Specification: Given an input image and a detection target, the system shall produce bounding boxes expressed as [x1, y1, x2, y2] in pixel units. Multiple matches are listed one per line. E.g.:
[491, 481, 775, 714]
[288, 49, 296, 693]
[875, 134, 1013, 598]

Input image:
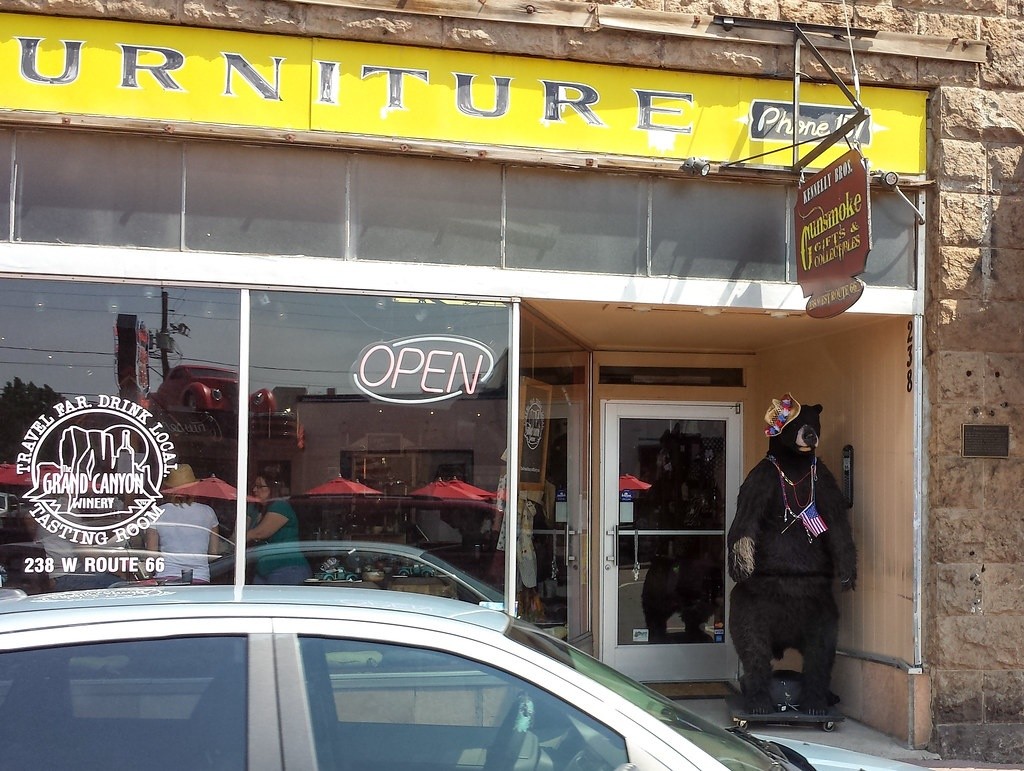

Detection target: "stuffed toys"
[728, 395, 855, 714]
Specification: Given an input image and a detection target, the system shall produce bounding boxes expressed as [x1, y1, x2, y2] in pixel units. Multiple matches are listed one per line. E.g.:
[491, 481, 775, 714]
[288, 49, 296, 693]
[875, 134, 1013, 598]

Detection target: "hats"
[162, 464, 196, 487]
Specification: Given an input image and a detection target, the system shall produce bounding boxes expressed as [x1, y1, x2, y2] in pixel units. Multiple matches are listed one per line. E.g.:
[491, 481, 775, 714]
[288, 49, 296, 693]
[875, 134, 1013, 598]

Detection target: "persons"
[226, 471, 312, 586]
[139, 464, 219, 585]
[497, 450, 544, 617]
[26, 474, 123, 592]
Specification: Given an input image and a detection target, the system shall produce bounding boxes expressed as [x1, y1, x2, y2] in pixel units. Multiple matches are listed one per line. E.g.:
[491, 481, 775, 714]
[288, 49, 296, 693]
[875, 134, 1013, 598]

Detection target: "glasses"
[253, 484, 269, 490]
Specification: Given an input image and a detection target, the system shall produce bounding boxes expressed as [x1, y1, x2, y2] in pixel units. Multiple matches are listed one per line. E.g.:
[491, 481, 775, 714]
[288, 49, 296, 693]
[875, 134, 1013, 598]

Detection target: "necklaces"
[764, 455, 818, 544]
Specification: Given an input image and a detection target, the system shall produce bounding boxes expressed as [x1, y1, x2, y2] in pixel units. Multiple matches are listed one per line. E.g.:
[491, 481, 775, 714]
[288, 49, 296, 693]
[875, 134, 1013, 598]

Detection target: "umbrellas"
[618, 474, 652, 490]
[160, 479, 259, 502]
[408, 480, 495, 500]
[0, 463, 101, 491]
[304, 478, 378, 495]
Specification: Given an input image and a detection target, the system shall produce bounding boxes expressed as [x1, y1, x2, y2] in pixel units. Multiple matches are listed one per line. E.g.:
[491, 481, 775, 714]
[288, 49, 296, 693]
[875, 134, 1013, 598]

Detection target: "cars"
[0, 492, 21, 514]
[0, 585, 931, 771]
[314, 567, 359, 582]
[149, 364, 277, 412]
[107, 539, 524, 621]
[397, 564, 438, 578]
[11, 502, 25, 510]
[1, 522, 236, 589]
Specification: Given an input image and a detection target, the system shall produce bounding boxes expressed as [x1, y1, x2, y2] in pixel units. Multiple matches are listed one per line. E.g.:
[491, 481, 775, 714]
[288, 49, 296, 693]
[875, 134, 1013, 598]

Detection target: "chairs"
[0, 657, 75, 771]
[0, 545, 50, 595]
[192, 661, 247, 771]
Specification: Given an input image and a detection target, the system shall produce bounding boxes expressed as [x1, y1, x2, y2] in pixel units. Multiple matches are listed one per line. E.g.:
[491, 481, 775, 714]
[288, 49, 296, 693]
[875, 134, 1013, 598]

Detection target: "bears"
[632, 428, 725, 645]
[727, 393, 859, 714]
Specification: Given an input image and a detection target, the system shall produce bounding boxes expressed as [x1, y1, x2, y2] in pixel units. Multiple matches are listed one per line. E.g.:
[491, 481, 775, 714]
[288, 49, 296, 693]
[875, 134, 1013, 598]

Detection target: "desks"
[301, 574, 457, 599]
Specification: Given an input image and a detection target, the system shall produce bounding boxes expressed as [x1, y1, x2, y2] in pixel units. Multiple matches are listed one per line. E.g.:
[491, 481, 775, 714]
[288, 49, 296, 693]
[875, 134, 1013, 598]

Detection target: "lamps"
[681, 156, 710, 178]
[874, 171, 901, 191]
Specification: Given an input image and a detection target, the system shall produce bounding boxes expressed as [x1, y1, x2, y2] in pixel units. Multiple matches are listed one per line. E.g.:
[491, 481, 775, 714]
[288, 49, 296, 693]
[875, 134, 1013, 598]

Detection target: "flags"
[800, 503, 828, 538]
